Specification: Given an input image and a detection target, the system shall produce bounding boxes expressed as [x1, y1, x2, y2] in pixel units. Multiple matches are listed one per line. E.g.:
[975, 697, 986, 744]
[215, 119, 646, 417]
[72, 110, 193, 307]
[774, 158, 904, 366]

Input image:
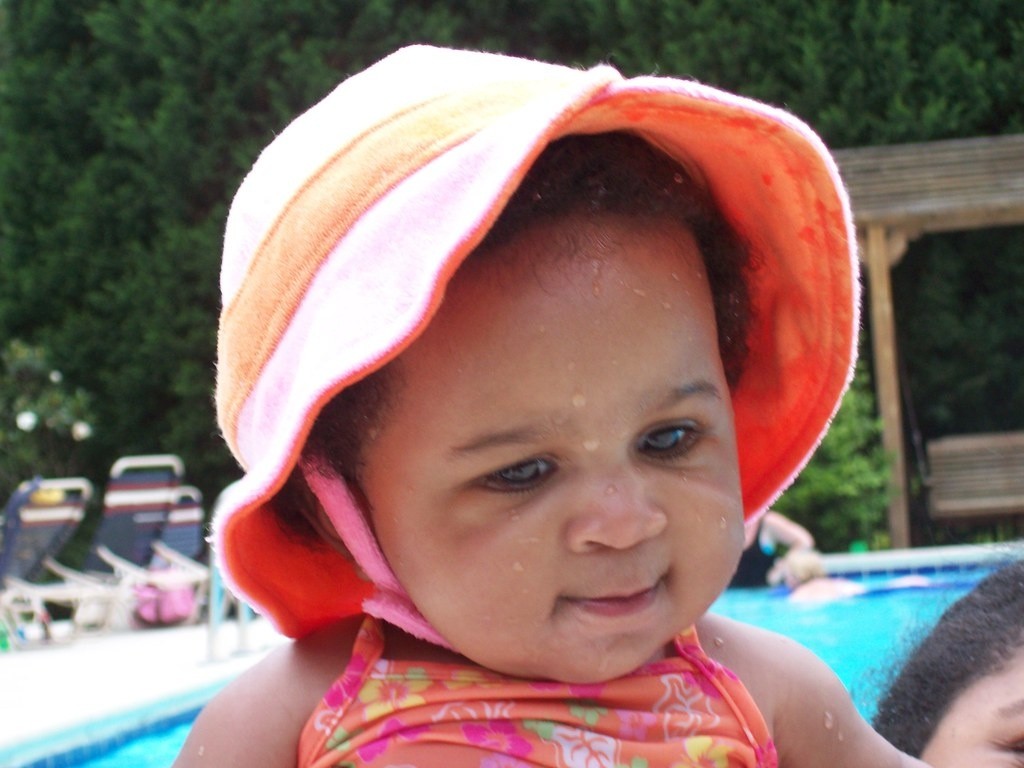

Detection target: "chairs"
[78, 453, 211, 633]
[164, 485, 205, 560]
[0, 477, 116, 652]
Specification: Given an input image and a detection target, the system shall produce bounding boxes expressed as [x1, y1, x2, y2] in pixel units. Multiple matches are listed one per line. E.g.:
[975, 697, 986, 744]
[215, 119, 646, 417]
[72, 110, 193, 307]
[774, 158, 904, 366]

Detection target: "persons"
[168, 43, 934, 768]
[727, 511, 929, 601]
[872, 560, 1024, 768]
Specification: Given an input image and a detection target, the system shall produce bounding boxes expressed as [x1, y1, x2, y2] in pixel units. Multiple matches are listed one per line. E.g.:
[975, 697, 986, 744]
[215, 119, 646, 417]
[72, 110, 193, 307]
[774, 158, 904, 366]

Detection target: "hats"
[210, 44, 861, 639]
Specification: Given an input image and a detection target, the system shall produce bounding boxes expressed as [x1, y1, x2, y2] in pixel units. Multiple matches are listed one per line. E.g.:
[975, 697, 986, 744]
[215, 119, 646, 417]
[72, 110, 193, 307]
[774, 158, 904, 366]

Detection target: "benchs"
[926, 430, 1024, 543]
